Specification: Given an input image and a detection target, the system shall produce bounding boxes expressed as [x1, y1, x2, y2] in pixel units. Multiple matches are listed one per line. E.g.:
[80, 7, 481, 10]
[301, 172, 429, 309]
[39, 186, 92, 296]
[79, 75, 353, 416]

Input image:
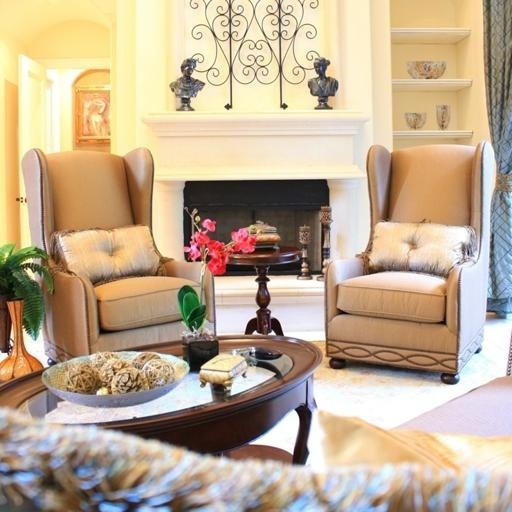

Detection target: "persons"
[307, 56, 339, 98]
[168, 58, 205, 100]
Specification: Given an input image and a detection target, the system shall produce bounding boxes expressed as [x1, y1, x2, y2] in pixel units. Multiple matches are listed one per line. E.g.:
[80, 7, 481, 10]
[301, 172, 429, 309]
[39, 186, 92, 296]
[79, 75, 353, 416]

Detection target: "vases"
[198, 262, 218, 341]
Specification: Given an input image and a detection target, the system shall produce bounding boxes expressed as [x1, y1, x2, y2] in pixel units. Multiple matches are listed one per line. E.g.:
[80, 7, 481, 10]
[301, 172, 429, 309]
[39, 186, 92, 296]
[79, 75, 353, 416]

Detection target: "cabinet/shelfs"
[390, 26, 473, 140]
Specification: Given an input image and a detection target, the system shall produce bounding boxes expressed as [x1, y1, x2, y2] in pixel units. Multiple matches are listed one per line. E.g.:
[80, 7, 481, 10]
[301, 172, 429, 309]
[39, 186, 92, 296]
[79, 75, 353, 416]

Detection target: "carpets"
[216, 303, 512, 470]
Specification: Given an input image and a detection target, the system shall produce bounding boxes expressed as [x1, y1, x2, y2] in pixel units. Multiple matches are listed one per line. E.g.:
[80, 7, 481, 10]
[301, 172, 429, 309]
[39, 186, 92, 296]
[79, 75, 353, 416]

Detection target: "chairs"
[18, 145, 216, 365]
[324, 140, 496, 386]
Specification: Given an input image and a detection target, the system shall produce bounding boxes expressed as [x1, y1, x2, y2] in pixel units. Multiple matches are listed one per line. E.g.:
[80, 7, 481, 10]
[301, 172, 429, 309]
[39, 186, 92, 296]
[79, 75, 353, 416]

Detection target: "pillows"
[50, 223, 167, 284]
[368, 220, 476, 278]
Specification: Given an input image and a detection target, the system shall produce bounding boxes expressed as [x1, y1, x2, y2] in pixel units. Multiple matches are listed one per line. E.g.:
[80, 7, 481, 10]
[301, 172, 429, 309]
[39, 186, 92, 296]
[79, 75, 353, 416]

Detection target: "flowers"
[178, 204, 257, 336]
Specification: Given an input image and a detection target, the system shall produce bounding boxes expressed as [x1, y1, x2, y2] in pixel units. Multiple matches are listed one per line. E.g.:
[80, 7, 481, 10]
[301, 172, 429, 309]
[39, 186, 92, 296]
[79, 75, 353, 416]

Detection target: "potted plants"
[0, 240, 55, 354]
[178, 285, 220, 373]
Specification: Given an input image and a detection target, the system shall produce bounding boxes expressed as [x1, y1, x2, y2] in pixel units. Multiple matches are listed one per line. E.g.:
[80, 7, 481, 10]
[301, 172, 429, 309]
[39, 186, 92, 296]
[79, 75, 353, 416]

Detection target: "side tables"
[228, 247, 302, 335]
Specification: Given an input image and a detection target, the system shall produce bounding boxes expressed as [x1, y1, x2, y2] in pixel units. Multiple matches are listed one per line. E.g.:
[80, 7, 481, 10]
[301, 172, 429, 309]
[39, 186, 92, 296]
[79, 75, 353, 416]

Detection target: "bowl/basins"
[40, 348, 191, 409]
